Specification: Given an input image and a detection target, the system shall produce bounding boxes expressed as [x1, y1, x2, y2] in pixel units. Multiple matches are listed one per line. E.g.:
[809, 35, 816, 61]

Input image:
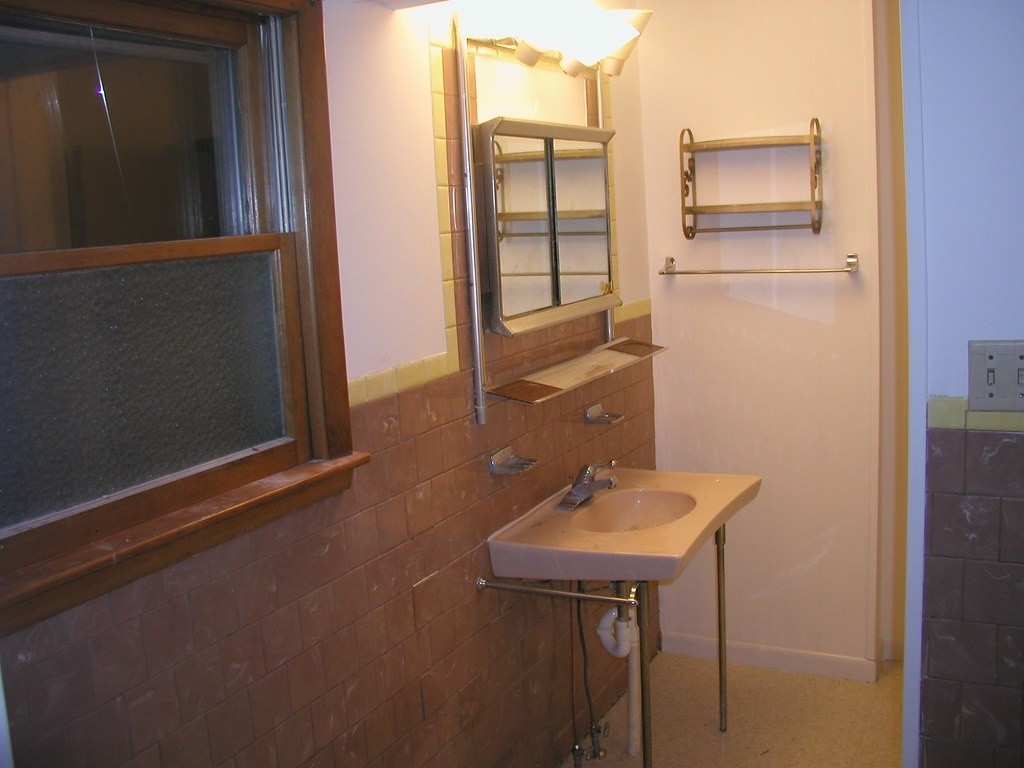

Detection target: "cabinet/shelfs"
[493, 141, 606, 242]
[679, 117, 822, 240]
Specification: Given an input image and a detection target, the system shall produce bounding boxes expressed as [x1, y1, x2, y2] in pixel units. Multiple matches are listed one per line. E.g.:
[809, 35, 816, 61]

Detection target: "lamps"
[515, 0, 654, 81]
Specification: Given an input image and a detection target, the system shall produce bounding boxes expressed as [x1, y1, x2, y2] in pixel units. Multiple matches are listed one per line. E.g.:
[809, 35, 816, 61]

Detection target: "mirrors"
[474, 115, 625, 338]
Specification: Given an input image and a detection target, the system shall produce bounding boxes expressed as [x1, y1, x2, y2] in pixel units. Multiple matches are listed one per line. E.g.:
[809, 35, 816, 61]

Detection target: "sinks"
[486, 466, 763, 581]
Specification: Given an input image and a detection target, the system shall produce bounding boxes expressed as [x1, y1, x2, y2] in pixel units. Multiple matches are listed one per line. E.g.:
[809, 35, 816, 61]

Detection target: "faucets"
[556, 460, 619, 512]
